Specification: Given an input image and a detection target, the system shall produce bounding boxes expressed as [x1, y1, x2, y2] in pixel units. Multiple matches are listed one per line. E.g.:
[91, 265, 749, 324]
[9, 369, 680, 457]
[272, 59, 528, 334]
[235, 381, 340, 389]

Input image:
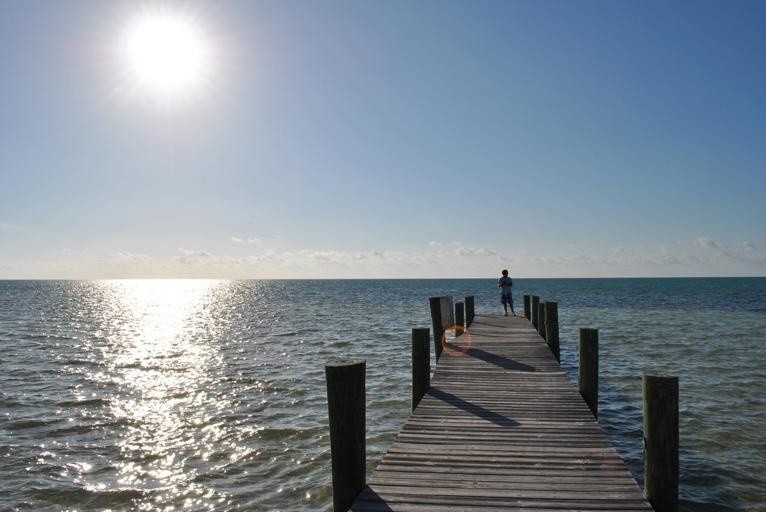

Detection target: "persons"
[497, 269, 516, 316]
